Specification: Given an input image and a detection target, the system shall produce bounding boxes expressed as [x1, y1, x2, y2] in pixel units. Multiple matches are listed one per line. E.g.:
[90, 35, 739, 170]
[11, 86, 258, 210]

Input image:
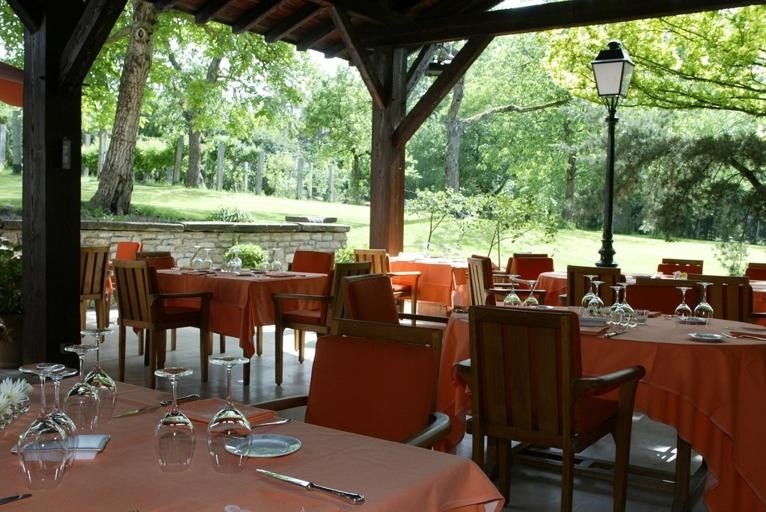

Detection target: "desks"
[442, 304, 766, 504]
[0, 363, 504, 512]
[152, 267, 328, 386]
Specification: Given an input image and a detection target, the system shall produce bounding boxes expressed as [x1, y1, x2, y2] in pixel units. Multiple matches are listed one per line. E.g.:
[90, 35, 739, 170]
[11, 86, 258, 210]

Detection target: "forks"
[721, 331, 765, 341]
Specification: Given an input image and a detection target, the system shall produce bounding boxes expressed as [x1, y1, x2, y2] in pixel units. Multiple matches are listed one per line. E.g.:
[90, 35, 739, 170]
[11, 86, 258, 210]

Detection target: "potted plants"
[0, 239, 26, 370]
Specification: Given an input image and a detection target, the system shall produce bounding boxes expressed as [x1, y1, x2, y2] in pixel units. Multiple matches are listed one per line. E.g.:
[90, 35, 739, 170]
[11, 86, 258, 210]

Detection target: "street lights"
[589, 39, 634, 267]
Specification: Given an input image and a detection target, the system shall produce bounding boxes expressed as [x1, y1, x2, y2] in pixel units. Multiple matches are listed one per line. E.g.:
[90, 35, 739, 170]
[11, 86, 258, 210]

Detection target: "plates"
[689, 332, 725, 343]
[223, 433, 302, 460]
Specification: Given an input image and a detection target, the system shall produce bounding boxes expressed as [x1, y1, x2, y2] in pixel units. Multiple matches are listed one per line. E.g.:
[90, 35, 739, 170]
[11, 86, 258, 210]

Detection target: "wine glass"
[578, 273, 638, 331]
[153, 366, 196, 474]
[190, 246, 282, 275]
[694, 282, 715, 329]
[205, 354, 253, 476]
[521, 281, 541, 307]
[503, 275, 523, 307]
[15, 327, 116, 491]
[674, 285, 693, 330]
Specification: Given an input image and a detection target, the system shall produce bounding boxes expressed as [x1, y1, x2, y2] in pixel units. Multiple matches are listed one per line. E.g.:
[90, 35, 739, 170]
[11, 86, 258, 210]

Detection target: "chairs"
[305, 320, 450, 452]
[457, 306, 646, 512]
[82, 242, 766, 387]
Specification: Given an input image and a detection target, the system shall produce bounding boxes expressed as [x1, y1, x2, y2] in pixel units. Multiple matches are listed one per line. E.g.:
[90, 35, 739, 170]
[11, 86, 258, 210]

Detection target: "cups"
[634, 307, 649, 325]
[680, 273, 687, 281]
[674, 272, 681, 280]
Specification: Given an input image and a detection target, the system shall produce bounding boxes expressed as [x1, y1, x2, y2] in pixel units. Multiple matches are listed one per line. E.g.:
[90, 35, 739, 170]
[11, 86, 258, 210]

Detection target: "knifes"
[596, 326, 626, 337]
[0, 494, 32, 506]
[111, 393, 201, 420]
[256, 467, 366, 506]
[182, 270, 218, 277]
